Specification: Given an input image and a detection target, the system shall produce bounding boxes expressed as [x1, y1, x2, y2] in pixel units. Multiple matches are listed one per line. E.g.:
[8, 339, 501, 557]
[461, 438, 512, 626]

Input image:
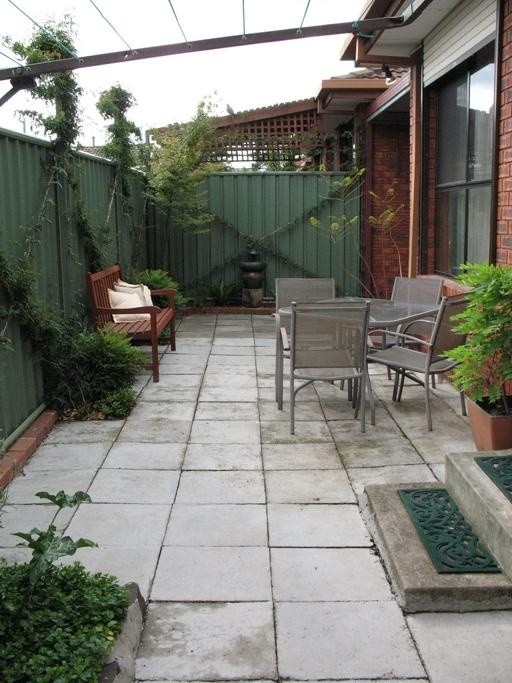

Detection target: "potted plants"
[441, 262, 512, 450]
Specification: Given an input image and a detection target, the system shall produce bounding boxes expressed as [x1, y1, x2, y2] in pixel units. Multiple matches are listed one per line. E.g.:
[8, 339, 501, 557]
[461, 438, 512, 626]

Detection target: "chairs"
[354, 275, 445, 388]
[277, 302, 368, 436]
[351, 290, 480, 434]
[275, 274, 347, 394]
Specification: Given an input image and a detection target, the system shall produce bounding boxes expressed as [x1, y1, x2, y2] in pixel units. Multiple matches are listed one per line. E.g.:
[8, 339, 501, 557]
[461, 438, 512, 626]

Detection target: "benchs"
[88, 262, 178, 382]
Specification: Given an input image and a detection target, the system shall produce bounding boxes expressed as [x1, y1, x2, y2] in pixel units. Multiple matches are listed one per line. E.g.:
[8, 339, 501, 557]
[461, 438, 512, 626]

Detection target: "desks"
[271, 293, 450, 412]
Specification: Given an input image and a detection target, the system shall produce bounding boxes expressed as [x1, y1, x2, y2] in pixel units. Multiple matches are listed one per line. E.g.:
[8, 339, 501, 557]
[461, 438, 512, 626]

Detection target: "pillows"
[106, 276, 156, 325]
